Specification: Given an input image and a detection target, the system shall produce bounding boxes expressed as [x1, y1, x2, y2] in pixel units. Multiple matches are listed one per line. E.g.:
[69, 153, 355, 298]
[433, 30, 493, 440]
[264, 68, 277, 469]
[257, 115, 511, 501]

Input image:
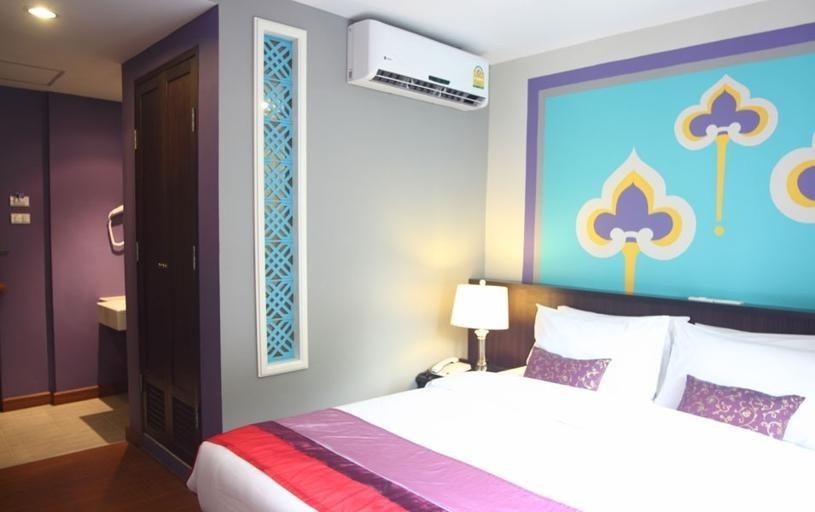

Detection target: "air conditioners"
[346, 19, 490, 112]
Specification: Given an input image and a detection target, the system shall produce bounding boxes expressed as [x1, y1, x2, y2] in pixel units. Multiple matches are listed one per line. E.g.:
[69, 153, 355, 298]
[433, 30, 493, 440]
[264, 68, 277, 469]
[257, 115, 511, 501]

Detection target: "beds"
[186, 280, 815, 512]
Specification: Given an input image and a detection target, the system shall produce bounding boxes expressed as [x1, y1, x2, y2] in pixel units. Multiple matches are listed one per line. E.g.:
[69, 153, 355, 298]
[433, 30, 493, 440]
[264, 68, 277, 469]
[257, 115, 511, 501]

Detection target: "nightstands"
[416, 356, 508, 388]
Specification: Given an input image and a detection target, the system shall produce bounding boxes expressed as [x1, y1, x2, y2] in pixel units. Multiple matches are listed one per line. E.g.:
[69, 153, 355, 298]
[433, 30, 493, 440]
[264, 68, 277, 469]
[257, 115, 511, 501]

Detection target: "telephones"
[430, 357, 472, 376]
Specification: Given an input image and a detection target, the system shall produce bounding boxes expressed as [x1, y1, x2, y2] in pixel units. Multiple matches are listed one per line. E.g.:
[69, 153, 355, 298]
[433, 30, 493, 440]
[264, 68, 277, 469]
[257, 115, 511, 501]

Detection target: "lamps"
[451, 280, 510, 372]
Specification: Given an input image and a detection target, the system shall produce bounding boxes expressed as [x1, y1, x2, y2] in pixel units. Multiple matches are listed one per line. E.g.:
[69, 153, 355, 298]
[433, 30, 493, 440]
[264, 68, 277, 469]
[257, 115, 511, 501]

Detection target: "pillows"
[526, 302, 671, 406]
[522, 346, 612, 393]
[692, 320, 815, 352]
[654, 320, 815, 448]
[675, 373, 805, 444]
[556, 303, 691, 397]
